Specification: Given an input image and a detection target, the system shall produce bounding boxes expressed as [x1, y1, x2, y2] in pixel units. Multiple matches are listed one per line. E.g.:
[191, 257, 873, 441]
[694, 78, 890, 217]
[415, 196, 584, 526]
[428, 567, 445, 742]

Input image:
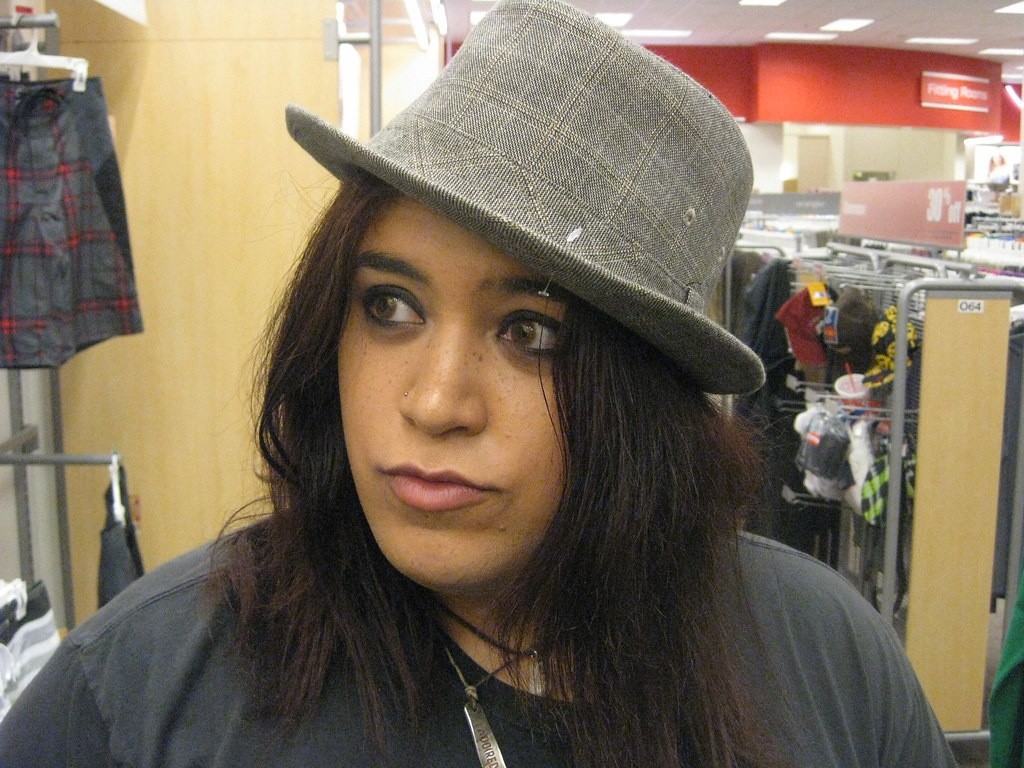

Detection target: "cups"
[834, 373, 872, 423]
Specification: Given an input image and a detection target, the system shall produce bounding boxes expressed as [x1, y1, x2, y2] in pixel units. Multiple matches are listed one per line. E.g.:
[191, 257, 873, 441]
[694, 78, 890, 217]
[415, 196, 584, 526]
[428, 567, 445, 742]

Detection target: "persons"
[0, 1, 965, 768]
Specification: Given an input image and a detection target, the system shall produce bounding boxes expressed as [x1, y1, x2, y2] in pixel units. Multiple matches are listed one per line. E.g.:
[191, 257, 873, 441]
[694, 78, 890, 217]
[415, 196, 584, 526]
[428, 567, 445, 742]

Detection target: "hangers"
[0, 577, 42, 638]
[108, 453, 128, 526]
[0, 11, 89, 93]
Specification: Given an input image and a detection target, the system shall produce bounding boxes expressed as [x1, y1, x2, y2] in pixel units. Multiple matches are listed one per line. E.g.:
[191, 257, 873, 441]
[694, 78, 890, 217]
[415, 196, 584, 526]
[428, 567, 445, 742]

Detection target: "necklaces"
[436, 601, 559, 767]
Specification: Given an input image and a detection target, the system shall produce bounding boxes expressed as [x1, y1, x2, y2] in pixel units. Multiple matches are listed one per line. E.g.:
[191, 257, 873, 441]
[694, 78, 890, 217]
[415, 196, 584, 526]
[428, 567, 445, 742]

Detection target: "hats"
[813, 291, 877, 373]
[864, 305, 917, 389]
[281, 1, 765, 394]
[773, 288, 839, 367]
